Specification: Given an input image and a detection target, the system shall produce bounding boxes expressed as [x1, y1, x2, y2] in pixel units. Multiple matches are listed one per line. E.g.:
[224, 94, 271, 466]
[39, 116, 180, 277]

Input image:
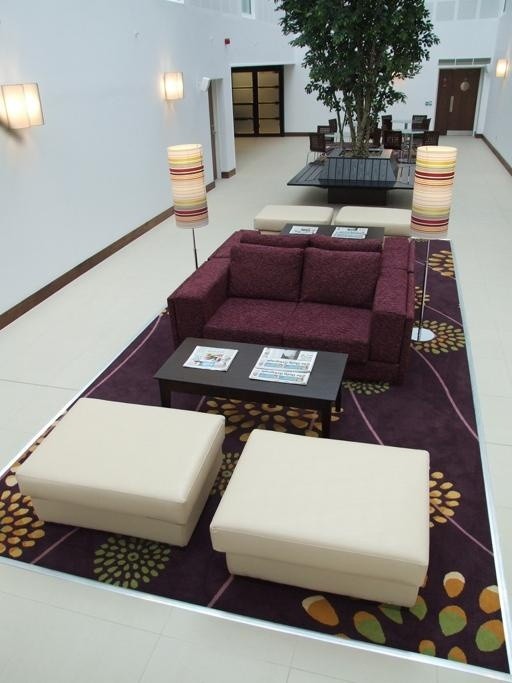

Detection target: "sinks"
[166, 242, 413, 383]
[208, 229, 416, 273]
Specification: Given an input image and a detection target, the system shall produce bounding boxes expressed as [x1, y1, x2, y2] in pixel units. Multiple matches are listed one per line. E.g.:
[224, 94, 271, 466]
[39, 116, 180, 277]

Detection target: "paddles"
[0, 235, 509, 681]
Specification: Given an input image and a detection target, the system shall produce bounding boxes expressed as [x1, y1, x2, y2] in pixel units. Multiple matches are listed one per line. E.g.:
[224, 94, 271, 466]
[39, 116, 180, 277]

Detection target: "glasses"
[164, 71, 184, 103]
[410, 145, 457, 340]
[166, 143, 210, 271]
[495, 59, 507, 78]
[0, 82, 45, 132]
[494, 58, 507, 80]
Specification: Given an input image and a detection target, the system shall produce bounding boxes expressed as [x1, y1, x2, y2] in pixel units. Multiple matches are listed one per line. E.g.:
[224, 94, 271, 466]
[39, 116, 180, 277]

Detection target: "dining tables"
[273, 0, 441, 160]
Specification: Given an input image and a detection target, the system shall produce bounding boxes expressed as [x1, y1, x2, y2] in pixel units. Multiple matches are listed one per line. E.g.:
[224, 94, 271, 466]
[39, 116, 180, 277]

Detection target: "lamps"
[305, 114, 439, 165]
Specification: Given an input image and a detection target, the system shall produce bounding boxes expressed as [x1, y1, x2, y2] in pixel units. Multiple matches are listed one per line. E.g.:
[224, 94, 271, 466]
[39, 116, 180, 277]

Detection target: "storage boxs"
[287, 149, 415, 204]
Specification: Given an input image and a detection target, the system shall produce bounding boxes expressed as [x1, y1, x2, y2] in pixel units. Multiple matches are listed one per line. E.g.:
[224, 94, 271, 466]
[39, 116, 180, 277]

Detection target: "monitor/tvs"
[331, 226, 368, 239]
[182, 345, 239, 372]
[289, 224, 319, 235]
[249, 347, 317, 385]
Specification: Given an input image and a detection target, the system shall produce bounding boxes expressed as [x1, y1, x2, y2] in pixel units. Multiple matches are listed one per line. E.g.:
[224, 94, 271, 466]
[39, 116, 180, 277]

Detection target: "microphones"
[254, 205, 333, 231]
[17, 397, 225, 550]
[334, 206, 412, 236]
[209, 428, 432, 611]
